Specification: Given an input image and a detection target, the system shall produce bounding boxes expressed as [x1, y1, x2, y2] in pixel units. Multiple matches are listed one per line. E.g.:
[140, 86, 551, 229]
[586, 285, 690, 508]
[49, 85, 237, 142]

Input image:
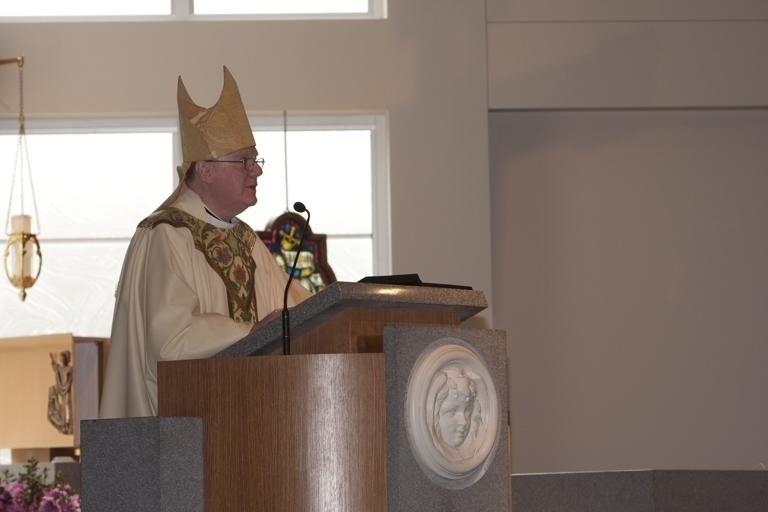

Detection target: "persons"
[98, 147, 314, 421]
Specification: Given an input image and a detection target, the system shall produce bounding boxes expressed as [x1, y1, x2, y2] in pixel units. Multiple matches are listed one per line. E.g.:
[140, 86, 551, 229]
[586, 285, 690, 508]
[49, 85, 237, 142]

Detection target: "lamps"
[0, 56, 42, 302]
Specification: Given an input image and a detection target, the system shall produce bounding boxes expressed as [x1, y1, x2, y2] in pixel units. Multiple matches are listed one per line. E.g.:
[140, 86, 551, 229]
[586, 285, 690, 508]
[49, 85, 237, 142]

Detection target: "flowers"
[2, 456, 80, 512]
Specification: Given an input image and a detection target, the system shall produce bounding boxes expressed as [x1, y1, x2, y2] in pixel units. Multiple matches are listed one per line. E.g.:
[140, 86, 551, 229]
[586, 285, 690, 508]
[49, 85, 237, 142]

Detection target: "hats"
[177, 64, 257, 164]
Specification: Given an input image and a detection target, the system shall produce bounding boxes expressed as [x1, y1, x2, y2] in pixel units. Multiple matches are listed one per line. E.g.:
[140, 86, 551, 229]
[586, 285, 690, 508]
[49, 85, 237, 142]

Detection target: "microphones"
[282, 201, 310, 355]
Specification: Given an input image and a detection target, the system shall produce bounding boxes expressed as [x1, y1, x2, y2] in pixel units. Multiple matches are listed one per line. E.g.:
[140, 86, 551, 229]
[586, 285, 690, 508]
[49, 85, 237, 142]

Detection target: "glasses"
[208, 155, 265, 172]
